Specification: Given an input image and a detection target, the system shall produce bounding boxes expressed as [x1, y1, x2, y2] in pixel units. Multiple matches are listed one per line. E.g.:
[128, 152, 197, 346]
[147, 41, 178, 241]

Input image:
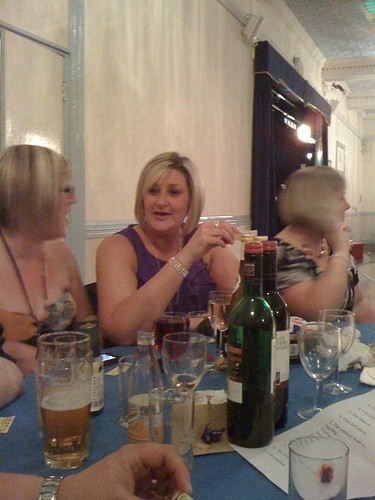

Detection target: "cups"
[119, 354, 154, 429]
[187, 309, 218, 371]
[34, 331, 94, 470]
[350, 241, 364, 264]
[288, 436, 349, 500]
[161, 332, 208, 399]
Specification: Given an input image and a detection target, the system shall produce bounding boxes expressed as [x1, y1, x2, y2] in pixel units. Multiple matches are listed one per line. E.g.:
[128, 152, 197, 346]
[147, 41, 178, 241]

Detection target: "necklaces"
[151, 234, 181, 309]
[299, 231, 326, 257]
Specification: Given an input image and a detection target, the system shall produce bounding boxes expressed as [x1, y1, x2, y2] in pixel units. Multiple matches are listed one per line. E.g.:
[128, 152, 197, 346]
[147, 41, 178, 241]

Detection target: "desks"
[0, 320, 375, 500]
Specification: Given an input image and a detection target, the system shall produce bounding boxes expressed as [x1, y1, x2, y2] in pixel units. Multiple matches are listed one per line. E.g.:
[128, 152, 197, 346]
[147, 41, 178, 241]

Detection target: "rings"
[151, 479, 158, 491]
[213, 221, 219, 227]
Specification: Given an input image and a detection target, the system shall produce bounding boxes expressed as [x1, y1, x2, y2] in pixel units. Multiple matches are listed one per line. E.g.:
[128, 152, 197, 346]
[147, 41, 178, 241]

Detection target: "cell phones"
[102, 353, 121, 366]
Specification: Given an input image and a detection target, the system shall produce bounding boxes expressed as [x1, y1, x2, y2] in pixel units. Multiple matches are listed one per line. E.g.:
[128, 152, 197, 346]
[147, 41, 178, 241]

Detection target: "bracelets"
[39, 475, 64, 500]
[168, 257, 188, 277]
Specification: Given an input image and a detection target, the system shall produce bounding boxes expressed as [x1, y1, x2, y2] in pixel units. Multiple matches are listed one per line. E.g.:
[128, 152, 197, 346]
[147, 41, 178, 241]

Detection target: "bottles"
[126, 330, 165, 444]
[226, 241, 276, 449]
[261, 241, 291, 430]
[77, 320, 105, 416]
[230, 233, 257, 309]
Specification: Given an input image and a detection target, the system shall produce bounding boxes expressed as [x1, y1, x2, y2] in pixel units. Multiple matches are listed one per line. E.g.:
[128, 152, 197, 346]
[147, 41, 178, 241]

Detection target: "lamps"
[241, 12, 265, 43]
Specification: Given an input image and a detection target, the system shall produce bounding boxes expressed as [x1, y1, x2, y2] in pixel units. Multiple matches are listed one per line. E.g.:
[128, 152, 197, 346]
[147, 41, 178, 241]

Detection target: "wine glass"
[154, 311, 190, 393]
[207, 289, 233, 373]
[316, 308, 356, 395]
[298, 322, 342, 421]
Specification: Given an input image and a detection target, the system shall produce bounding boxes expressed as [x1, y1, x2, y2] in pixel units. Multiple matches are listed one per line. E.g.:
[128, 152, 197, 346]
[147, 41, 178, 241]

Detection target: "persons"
[0, 442, 191, 500]
[270, 166, 375, 324]
[95, 152, 242, 346]
[0, 143, 95, 409]
